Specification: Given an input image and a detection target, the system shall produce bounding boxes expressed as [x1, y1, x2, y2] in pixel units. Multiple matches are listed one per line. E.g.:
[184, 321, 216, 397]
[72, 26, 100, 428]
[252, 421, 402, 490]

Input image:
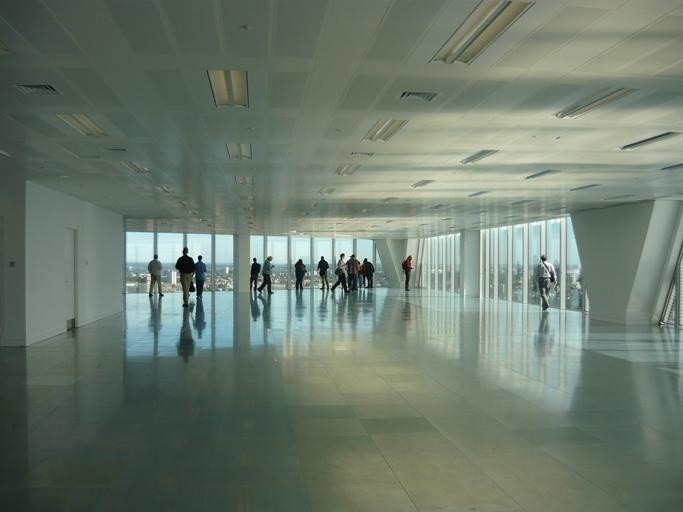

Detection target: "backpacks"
[402, 260, 406, 270]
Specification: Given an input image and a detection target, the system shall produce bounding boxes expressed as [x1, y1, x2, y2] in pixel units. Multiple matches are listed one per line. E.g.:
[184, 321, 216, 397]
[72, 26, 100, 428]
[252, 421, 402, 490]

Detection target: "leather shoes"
[320, 286, 374, 293]
[149, 294, 153, 296]
[159, 293, 164, 296]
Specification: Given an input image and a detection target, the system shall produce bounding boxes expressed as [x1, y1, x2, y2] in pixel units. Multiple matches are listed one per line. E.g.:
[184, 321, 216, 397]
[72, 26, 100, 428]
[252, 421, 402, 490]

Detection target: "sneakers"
[258, 288, 262, 293]
[268, 292, 274, 294]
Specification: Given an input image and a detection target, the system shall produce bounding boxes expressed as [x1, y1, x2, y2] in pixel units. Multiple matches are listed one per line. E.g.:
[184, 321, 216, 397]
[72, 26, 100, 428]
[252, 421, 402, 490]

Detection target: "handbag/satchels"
[189, 282, 195, 292]
[335, 268, 342, 275]
[550, 274, 554, 282]
[320, 270, 325, 276]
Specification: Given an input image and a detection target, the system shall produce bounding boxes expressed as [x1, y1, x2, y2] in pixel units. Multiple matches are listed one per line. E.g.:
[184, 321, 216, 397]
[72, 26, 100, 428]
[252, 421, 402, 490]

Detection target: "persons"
[402, 255, 416, 291]
[250, 256, 274, 294]
[331, 253, 374, 293]
[536, 254, 557, 310]
[148, 254, 164, 296]
[316, 256, 329, 289]
[294, 259, 307, 289]
[175, 247, 207, 307]
